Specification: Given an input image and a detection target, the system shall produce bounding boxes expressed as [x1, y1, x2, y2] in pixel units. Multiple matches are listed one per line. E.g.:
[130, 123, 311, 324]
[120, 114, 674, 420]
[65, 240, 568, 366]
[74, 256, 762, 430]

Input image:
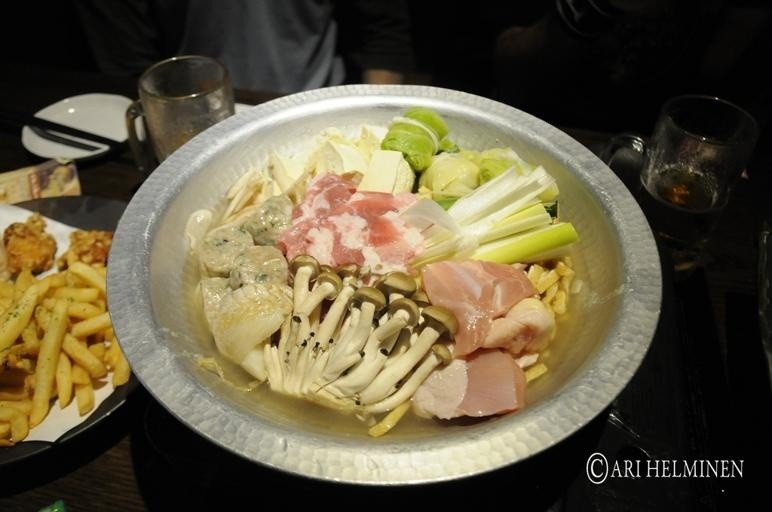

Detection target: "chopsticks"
[0, 102, 130, 152]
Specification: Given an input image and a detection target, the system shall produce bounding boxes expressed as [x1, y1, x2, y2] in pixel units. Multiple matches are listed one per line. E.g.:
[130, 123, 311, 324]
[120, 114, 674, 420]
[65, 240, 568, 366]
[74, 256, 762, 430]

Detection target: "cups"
[598, 94, 760, 272]
[125, 54, 235, 178]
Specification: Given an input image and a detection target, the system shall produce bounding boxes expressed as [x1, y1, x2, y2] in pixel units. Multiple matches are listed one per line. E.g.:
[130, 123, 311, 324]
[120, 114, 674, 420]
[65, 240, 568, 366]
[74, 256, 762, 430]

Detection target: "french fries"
[0, 242, 132, 447]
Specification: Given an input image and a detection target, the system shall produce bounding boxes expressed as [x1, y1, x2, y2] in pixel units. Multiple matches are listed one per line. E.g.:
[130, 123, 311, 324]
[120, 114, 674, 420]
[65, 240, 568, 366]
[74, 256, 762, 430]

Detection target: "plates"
[21, 92, 141, 159]
[0, 194, 139, 467]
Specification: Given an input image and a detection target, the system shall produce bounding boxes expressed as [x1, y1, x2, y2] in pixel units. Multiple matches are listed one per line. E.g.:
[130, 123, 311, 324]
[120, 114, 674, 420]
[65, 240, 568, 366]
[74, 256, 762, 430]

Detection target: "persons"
[176, 0, 585, 109]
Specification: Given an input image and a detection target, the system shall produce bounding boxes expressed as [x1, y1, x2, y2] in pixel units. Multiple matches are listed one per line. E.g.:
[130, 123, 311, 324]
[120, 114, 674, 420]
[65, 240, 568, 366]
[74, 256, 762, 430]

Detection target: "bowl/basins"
[105, 87, 664, 487]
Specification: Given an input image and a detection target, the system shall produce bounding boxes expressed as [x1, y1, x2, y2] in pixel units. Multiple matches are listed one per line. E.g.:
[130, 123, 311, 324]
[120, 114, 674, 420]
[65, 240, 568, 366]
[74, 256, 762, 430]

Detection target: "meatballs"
[201, 227, 254, 277]
[228, 245, 289, 291]
[243, 195, 294, 247]
